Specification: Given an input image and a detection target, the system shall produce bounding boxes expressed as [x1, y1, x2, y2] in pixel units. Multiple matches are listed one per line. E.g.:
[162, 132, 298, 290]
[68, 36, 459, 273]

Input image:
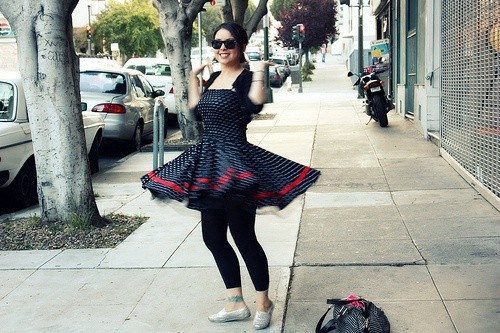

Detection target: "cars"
[189, 44, 212, 59]
[245, 46, 263, 61]
[209, 62, 222, 76]
[78, 65, 169, 154]
[268, 65, 284, 88]
[0, 67, 105, 212]
[122, 55, 207, 130]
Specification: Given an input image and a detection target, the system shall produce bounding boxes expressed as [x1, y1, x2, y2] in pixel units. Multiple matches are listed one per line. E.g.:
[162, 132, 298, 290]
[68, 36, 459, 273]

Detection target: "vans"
[79, 56, 120, 70]
[272, 51, 297, 66]
[253, 34, 272, 43]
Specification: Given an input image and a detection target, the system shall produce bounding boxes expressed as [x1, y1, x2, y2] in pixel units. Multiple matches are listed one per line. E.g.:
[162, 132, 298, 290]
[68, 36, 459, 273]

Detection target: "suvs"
[269, 56, 291, 77]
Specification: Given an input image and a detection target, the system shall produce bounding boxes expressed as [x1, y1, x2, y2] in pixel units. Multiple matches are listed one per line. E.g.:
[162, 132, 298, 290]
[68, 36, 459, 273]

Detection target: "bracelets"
[252, 70, 265, 73]
[251, 80, 264, 82]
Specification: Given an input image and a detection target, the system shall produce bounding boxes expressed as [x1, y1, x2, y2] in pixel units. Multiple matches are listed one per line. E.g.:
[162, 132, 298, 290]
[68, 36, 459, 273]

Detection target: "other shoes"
[208, 305, 251, 323]
[253, 303, 275, 329]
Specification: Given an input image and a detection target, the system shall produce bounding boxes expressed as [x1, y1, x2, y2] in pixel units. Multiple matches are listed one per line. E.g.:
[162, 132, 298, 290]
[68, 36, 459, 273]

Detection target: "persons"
[320, 45, 327, 63]
[139, 22, 321, 331]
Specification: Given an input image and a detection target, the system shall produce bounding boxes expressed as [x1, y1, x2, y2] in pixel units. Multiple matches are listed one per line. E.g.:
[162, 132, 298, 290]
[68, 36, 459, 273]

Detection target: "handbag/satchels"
[315, 295, 390, 333]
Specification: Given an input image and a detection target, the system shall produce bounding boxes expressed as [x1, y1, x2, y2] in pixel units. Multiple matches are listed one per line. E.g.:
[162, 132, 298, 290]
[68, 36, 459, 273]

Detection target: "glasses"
[211, 39, 239, 49]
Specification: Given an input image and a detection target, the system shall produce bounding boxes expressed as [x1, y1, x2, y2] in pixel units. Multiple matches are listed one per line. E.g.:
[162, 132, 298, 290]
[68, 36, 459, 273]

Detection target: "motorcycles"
[347, 57, 396, 128]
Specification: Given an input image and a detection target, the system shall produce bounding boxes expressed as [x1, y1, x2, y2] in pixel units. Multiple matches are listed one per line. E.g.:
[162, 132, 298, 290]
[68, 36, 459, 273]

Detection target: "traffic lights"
[291, 26, 299, 41]
[86, 25, 92, 40]
[297, 23, 305, 42]
